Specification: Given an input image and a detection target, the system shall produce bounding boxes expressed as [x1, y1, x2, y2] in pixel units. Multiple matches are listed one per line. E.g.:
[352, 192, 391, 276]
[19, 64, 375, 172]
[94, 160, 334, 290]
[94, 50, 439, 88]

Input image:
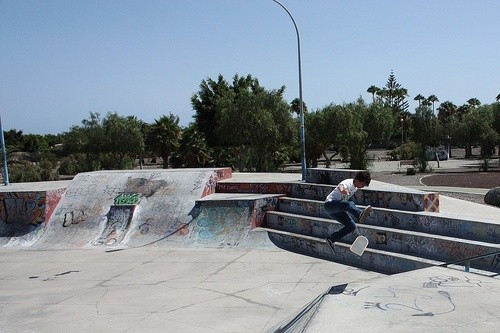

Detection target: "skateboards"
[348, 235, 369, 259]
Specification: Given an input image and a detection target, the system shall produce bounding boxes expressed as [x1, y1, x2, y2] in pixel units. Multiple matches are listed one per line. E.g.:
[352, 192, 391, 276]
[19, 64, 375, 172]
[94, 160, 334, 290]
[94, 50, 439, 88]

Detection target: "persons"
[324, 170, 372, 254]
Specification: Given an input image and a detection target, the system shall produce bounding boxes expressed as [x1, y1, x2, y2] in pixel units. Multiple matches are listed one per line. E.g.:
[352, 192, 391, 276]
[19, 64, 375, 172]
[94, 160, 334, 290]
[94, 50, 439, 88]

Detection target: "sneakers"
[358, 206, 372, 223]
[325, 237, 336, 255]
